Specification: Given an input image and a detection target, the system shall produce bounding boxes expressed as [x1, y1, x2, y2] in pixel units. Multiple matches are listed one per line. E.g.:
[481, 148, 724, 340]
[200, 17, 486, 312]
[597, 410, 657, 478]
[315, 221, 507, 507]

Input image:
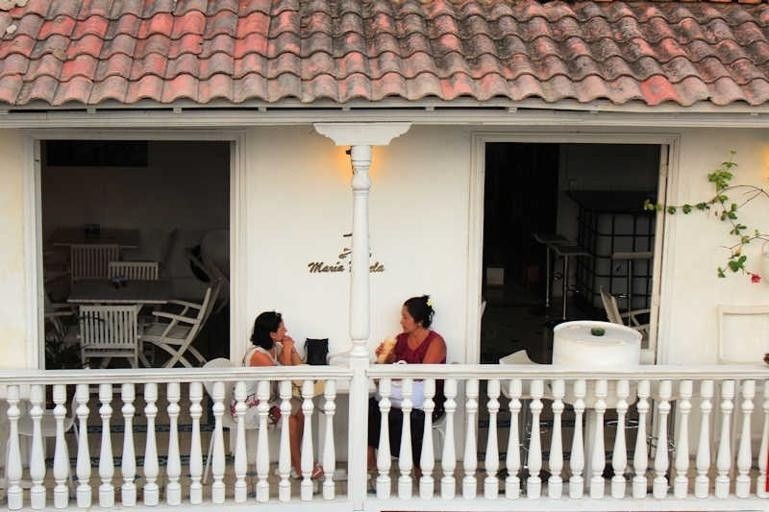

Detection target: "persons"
[230, 312, 324, 480]
[366, 295, 447, 492]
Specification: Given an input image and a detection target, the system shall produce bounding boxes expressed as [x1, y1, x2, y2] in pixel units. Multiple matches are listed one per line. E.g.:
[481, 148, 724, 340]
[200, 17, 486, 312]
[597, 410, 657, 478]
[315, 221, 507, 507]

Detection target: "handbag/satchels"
[376, 360, 424, 408]
[304, 337, 330, 366]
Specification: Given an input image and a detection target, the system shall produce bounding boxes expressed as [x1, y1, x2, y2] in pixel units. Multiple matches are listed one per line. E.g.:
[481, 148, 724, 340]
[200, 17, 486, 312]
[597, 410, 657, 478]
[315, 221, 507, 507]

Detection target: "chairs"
[494, 286, 676, 487]
[433, 360, 462, 473]
[42, 243, 221, 369]
[198, 357, 282, 484]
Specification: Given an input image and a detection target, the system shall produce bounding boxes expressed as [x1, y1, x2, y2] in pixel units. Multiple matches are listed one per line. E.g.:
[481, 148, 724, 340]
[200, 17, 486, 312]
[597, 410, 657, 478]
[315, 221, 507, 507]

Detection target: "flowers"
[642, 149, 769, 284]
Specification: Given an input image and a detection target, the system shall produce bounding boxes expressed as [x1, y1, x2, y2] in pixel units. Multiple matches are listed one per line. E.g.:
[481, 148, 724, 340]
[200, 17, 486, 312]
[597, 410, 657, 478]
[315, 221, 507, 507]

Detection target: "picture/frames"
[45, 140, 151, 171]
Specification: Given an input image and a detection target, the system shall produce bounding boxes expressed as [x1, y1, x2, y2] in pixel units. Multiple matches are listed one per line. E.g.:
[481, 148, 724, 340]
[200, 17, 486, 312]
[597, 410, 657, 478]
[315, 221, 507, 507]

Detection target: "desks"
[612, 250, 654, 326]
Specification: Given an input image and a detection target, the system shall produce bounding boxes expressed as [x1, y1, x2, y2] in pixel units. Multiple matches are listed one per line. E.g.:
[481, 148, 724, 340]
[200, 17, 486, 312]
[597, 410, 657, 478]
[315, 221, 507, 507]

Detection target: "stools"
[535, 231, 594, 321]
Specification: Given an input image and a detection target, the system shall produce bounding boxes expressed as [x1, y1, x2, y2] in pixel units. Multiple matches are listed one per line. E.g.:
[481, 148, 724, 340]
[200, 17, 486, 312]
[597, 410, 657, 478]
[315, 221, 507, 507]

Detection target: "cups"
[376, 336, 397, 365]
